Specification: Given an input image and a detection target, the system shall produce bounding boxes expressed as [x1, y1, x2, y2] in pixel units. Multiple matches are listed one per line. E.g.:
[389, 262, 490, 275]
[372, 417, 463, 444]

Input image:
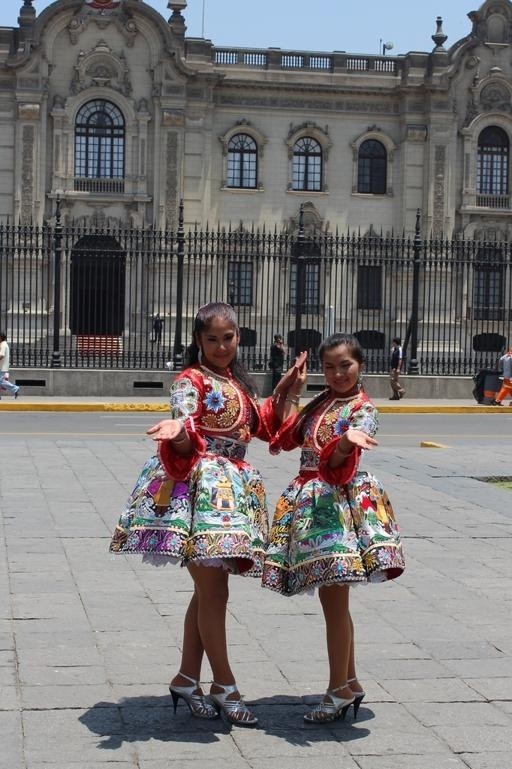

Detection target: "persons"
[389, 338, 406, 400]
[107, 302, 308, 727]
[153, 314, 164, 343]
[491, 347, 512, 407]
[269, 334, 286, 392]
[268, 333, 406, 724]
[0, 333, 20, 400]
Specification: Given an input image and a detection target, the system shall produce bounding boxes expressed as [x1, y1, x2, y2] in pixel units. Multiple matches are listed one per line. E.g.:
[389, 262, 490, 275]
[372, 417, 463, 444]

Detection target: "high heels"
[208, 680, 259, 726]
[304, 678, 365, 724]
[169, 671, 220, 719]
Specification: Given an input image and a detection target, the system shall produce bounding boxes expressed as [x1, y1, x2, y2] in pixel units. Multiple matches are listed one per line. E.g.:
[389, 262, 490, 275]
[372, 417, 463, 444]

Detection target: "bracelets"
[285, 392, 301, 404]
[171, 434, 186, 445]
[272, 386, 285, 399]
[336, 443, 354, 458]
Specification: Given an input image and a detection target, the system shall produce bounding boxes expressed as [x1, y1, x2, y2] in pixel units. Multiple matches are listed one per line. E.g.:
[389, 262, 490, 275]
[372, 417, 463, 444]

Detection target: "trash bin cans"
[477, 369, 504, 406]
